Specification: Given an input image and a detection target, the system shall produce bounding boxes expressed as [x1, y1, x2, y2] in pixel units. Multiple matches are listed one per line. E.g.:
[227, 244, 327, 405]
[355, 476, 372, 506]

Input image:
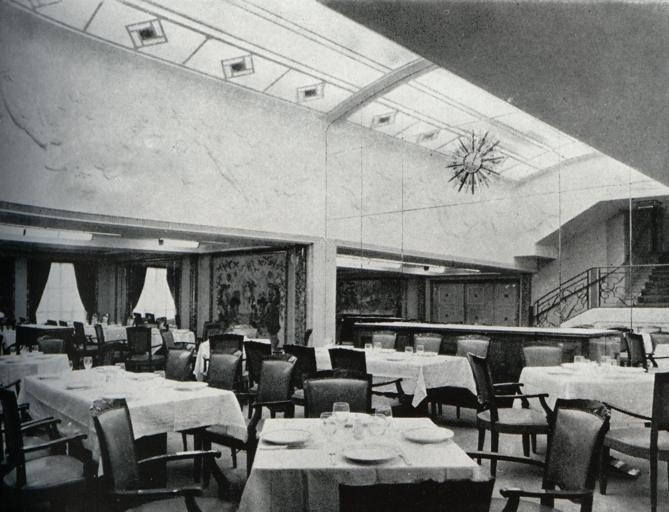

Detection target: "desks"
[2, 352, 72, 396]
[511, 362, 655, 459]
[18, 364, 248, 484]
[313, 345, 479, 415]
[353, 321, 623, 360]
[239, 416, 492, 510]
[82, 323, 131, 341]
[21, 320, 75, 331]
[146, 326, 196, 346]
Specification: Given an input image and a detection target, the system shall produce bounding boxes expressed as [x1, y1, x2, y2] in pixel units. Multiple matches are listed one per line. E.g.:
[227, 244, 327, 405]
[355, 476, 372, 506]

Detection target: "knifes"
[257, 445, 309, 451]
[397, 447, 414, 467]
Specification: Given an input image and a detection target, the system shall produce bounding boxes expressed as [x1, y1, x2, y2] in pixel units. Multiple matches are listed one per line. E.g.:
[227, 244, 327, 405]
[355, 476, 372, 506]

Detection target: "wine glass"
[83, 356, 93, 375]
[317, 401, 397, 451]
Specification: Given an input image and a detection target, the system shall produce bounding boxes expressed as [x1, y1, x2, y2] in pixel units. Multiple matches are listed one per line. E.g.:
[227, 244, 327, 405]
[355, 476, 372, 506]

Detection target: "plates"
[259, 427, 314, 446]
[175, 380, 209, 392]
[618, 367, 646, 374]
[128, 371, 159, 381]
[97, 364, 120, 373]
[36, 375, 134, 402]
[403, 426, 455, 443]
[560, 362, 590, 369]
[340, 442, 401, 462]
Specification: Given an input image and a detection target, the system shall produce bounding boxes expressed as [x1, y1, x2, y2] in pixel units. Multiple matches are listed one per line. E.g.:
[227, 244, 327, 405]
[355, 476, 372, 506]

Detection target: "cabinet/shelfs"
[432, 278, 520, 326]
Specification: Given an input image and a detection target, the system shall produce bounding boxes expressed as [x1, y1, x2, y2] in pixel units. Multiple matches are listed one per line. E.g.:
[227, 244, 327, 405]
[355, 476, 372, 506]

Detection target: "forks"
[328, 448, 337, 467]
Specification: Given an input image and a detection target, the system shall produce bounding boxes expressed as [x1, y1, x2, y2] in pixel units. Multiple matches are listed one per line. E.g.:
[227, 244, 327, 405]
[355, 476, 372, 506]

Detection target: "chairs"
[466, 351, 557, 465]
[2, 391, 98, 511]
[2, 315, 375, 422]
[600, 371, 667, 512]
[571, 314, 668, 370]
[88, 395, 239, 511]
[201, 399, 297, 478]
[412, 332, 443, 414]
[372, 329, 398, 350]
[498, 399, 611, 510]
[437, 334, 494, 417]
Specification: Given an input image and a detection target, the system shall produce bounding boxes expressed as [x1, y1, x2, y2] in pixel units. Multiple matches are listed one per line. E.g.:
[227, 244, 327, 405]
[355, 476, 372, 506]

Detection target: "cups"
[154, 369, 166, 387]
[116, 364, 126, 378]
[363, 341, 438, 361]
[572, 354, 617, 379]
[7, 344, 40, 358]
